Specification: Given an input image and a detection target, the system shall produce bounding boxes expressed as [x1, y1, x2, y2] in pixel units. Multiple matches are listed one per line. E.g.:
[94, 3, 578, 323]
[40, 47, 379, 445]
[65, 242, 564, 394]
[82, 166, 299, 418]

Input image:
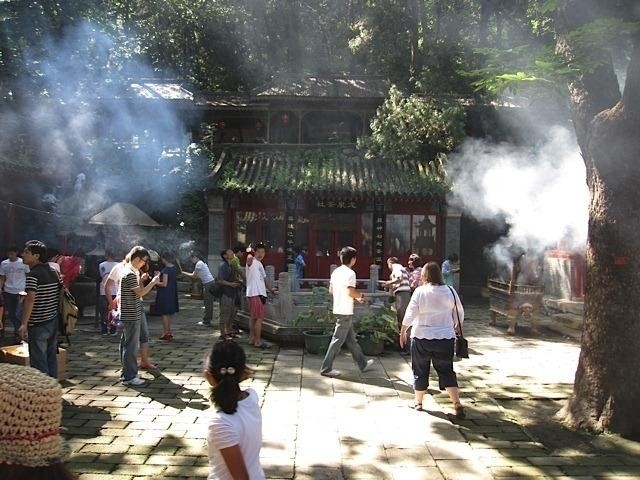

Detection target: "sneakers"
[220, 328, 243, 341]
[400, 351, 410, 355]
[362, 359, 374, 372]
[159, 334, 173, 339]
[198, 322, 209, 326]
[321, 369, 341, 376]
[103, 332, 118, 336]
[119, 374, 145, 385]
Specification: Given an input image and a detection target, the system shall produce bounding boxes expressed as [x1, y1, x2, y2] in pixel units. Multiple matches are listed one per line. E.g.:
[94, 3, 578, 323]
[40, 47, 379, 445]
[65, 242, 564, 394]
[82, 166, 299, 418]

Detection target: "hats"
[0, 363, 71, 467]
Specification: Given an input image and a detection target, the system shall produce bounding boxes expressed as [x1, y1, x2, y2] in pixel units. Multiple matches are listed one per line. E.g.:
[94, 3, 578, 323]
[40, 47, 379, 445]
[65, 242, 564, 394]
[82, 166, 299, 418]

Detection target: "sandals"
[408, 402, 422, 410]
[455, 402, 466, 419]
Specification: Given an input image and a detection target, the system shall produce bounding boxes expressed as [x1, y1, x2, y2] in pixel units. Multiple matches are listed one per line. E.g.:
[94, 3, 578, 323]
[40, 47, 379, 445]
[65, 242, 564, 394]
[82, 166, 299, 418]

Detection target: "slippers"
[140, 364, 157, 370]
[255, 343, 271, 348]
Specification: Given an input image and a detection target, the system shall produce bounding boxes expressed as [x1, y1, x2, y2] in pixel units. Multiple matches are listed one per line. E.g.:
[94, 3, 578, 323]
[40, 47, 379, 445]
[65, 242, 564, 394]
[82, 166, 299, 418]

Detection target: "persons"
[0, 361, 85, 479]
[382, 251, 460, 351]
[201, 337, 270, 480]
[218, 244, 306, 349]
[397, 260, 466, 421]
[2, 240, 84, 378]
[100, 246, 215, 384]
[321, 246, 374, 376]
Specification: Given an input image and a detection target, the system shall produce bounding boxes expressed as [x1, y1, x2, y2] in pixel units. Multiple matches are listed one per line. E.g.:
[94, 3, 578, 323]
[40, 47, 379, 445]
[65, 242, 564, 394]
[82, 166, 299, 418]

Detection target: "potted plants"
[352, 302, 401, 355]
[292, 286, 337, 358]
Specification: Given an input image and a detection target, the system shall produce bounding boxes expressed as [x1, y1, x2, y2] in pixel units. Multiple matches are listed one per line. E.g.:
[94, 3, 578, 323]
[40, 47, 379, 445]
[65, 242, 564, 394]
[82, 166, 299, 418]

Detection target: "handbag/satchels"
[107, 309, 123, 328]
[455, 335, 468, 358]
[209, 283, 225, 297]
[58, 284, 79, 335]
